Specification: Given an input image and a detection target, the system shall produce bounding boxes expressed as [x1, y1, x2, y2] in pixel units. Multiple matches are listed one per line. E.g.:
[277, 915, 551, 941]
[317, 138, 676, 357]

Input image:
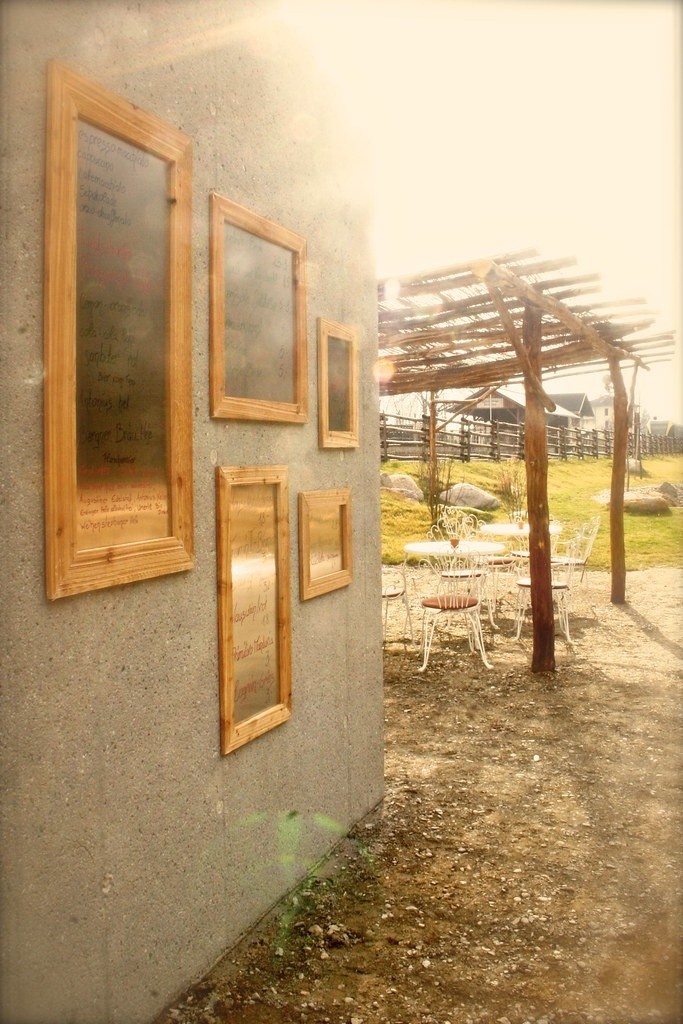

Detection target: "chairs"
[379, 505, 604, 673]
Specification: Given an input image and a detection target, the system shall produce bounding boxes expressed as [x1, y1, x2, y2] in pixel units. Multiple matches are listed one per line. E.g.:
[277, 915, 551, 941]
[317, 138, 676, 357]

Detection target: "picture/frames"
[316, 313, 360, 449]
[37, 63, 198, 609]
[299, 487, 352, 604]
[207, 190, 310, 425]
[214, 462, 292, 753]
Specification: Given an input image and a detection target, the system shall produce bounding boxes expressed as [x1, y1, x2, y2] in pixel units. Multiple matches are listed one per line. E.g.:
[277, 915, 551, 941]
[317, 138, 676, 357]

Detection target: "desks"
[402, 540, 505, 629]
[480, 520, 563, 536]
